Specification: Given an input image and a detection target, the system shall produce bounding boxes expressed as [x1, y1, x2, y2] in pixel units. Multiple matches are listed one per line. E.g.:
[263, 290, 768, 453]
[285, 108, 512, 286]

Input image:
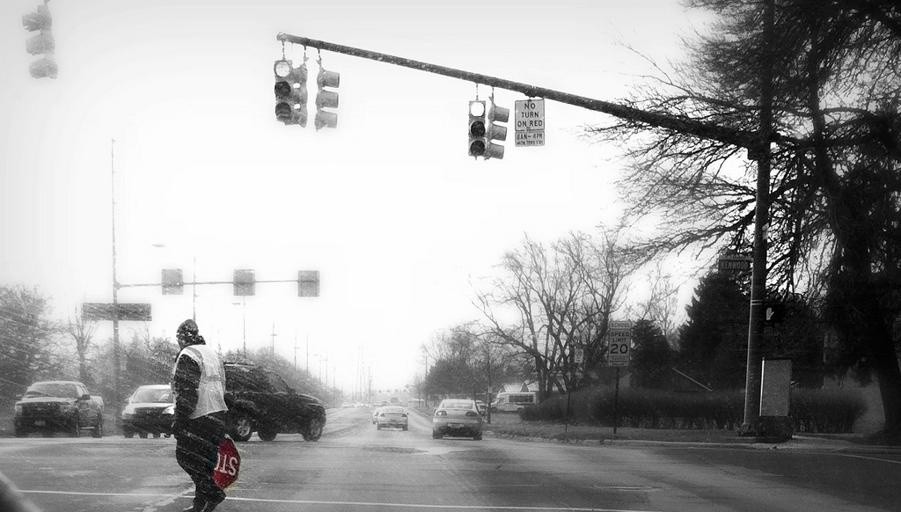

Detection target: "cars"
[13, 380, 105, 440]
[121, 385, 174, 439]
[375, 407, 409, 431]
[431, 399, 485, 442]
[340, 398, 387, 409]
[220, 358, 327, 443]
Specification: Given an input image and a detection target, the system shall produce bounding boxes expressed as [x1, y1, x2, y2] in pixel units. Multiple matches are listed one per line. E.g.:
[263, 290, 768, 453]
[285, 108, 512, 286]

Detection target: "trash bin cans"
[760, 358, 791, 441]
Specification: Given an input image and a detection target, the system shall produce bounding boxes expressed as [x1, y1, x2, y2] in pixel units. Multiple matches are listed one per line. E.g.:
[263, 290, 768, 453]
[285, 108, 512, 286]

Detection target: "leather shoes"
[182, 486, 226, 512]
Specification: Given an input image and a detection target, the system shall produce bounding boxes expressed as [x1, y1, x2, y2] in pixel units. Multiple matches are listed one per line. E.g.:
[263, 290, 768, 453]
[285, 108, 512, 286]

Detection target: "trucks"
[489, 390, 534, 416]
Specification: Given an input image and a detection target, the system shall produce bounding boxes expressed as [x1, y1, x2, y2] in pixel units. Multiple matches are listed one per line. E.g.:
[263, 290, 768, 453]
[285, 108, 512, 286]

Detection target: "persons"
[170, 319, 229, 511]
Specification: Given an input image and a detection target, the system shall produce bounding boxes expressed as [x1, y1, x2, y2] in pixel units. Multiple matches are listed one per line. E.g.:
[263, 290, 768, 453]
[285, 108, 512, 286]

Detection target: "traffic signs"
[718, 255, 753, 277]
[608, 320, 631, 368]
[514, 99, 545, 146]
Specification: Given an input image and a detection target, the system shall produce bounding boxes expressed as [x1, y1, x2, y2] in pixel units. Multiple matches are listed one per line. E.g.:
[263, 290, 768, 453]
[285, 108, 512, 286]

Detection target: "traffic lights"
[484, 105, 509, 159]
[314, 69, 339, 131]
[274, 60, 306, 131]
[469, 99, 485, 160]
